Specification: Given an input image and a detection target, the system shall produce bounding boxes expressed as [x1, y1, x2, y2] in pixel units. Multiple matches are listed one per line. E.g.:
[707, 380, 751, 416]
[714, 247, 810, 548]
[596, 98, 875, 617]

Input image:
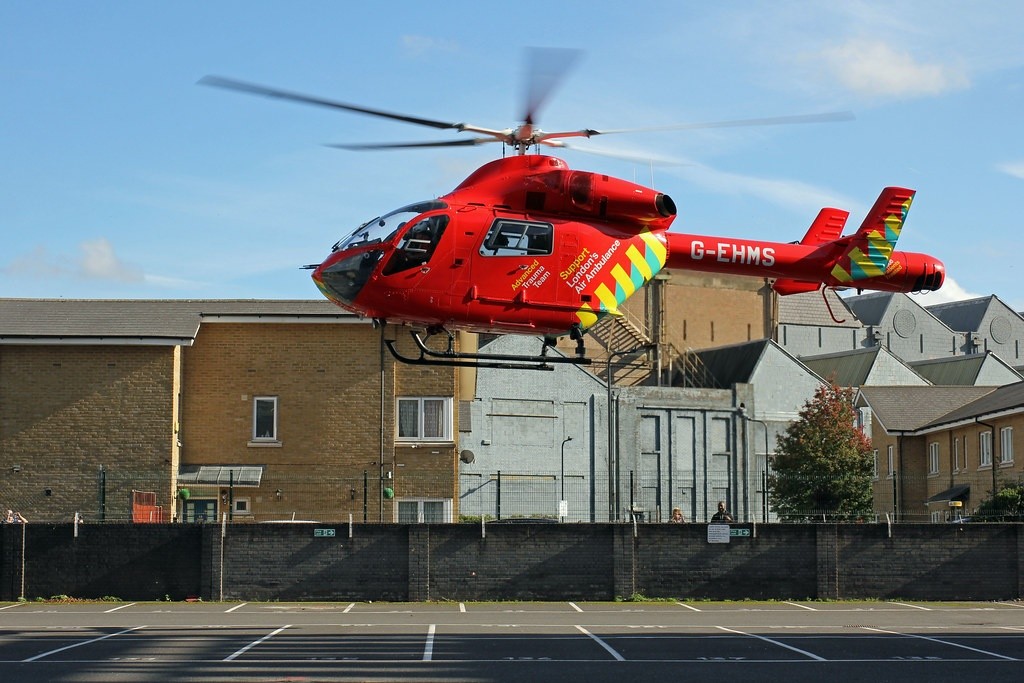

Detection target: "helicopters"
[195, 45, 948, 373]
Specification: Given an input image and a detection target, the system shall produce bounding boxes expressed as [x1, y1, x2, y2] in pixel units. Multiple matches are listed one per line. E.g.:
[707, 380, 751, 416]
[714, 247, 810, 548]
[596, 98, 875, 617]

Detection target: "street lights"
[741, 414, 769, 523]
[561, 436, 573, 522]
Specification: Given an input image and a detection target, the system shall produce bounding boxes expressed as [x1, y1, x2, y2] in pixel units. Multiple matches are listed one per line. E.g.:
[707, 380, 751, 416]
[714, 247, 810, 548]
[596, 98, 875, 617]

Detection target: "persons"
[0, 509, 29, 524]
[668, 508, 686, 524]
[710, 501, 734, 523]
[397, 218, 432, 250]
[72, 515, 84, 524]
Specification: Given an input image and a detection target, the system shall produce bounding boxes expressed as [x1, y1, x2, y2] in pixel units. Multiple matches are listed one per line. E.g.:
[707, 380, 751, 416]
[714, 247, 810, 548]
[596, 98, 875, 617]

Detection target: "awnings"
[177, 463, 263, 487]
[928, 484, 971, 504]
